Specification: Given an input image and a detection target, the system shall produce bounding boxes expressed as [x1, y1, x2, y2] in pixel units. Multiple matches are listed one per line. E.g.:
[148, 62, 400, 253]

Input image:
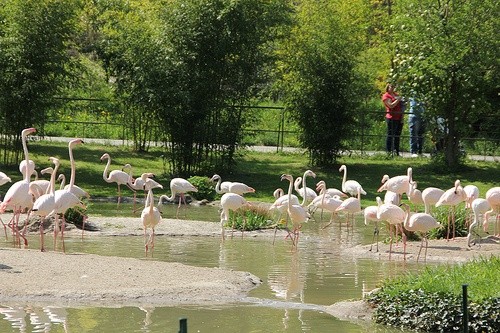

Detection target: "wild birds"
[0, 127, 500, 267]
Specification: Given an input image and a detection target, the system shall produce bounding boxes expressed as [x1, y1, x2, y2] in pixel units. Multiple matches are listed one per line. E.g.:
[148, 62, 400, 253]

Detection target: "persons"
[382, 83, 404, 156]
[409, 89, 425, 154]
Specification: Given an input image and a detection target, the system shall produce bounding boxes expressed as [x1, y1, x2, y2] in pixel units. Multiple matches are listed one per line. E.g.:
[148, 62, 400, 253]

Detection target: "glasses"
[389, 85, 395, 88]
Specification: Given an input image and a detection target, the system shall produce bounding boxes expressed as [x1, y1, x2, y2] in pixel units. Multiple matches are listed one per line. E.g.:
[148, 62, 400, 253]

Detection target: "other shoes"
[411, 154, 418, 157]
